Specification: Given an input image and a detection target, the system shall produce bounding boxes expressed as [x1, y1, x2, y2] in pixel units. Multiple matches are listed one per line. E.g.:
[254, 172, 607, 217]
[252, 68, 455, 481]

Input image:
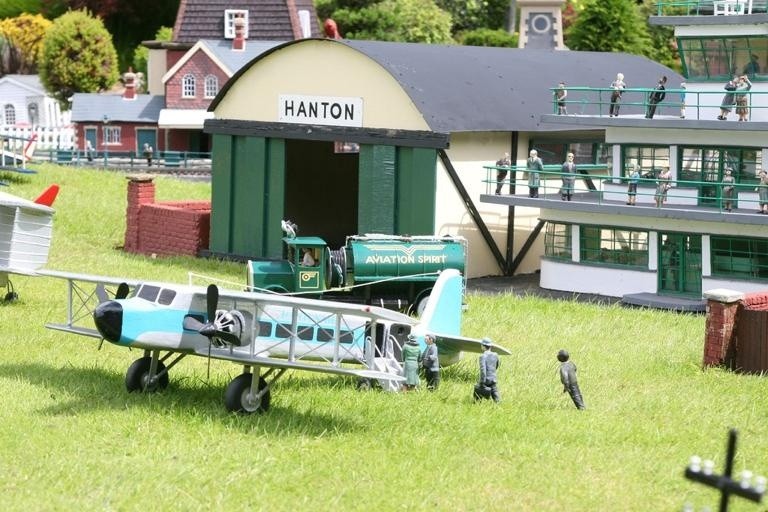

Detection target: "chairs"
[714, 0, 752, 16]
